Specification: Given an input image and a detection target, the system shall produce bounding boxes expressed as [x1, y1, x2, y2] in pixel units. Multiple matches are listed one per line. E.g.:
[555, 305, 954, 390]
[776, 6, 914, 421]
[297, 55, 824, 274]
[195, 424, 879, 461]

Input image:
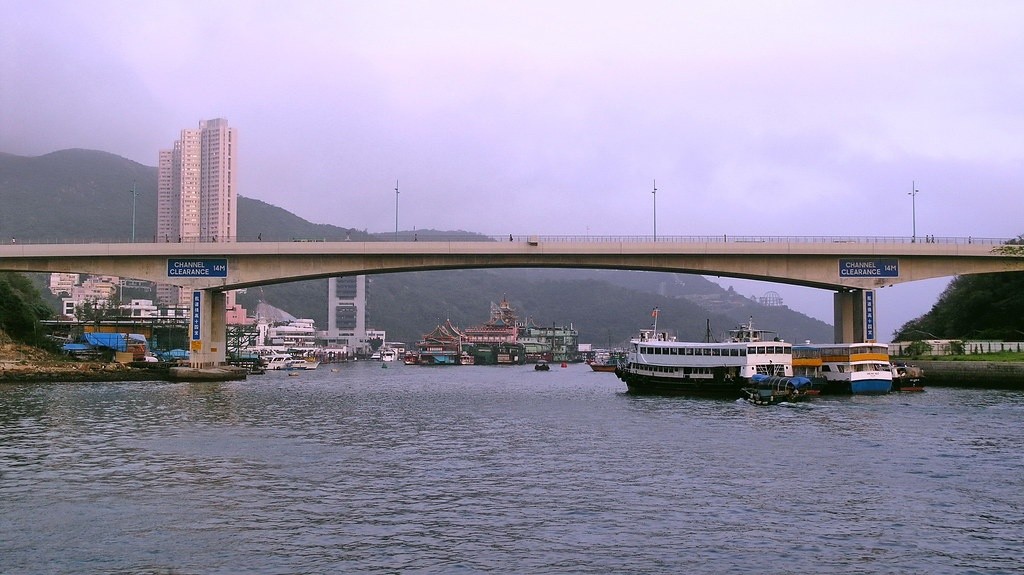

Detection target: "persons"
[510, 234, 513, 241]
[926, 235, 929, 243]
[968, 236, 972, 243]
[178, 235, 181, 243]
[414, 233, 418, 240]
[258, 233, 262, 241]
[12, 237, 16, 244]
[931, 235, 935, 243]
[212, 235, 217, 243]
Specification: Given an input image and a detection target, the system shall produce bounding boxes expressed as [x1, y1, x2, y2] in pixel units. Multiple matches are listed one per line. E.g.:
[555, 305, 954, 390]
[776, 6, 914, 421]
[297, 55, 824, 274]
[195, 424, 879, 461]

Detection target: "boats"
[889, 361, 923, 391]
[262, 354, 293, 369]
[791, 342, 893, 398]
[587, 358, 618, 372]
[616, 336, 794, 395]
[284, 358, 321, 370]
[535, 359, 552, 372]
[727, 372, 812, 404]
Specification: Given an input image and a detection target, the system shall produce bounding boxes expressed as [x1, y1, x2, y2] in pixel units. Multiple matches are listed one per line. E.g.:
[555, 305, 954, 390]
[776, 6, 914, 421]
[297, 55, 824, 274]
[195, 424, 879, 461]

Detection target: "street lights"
[127, 178, 140, 243]
[393, 178, 402, 241]
[651, 178, 660, 241]
